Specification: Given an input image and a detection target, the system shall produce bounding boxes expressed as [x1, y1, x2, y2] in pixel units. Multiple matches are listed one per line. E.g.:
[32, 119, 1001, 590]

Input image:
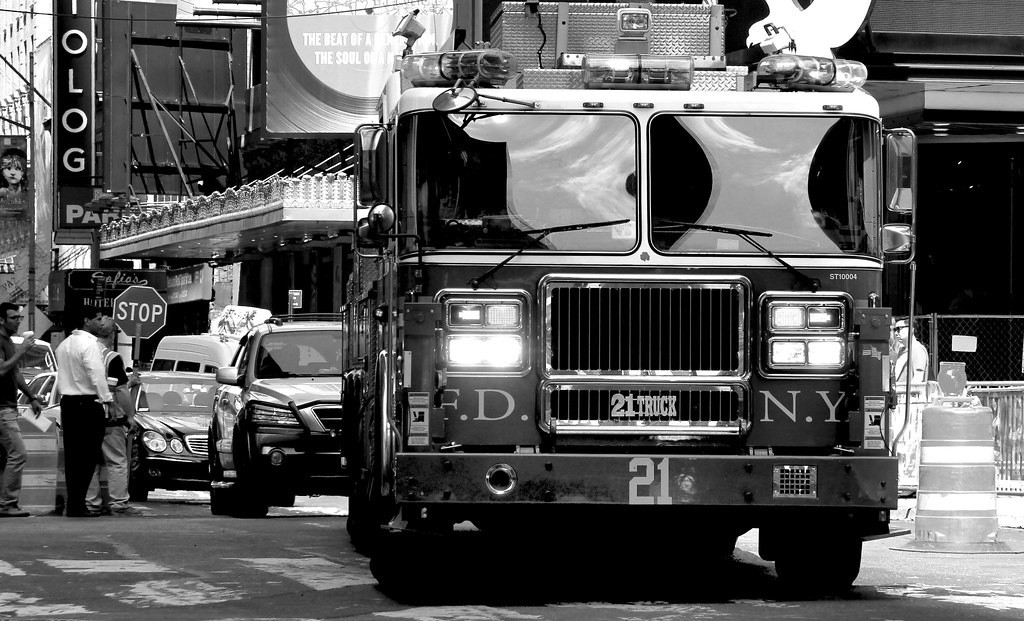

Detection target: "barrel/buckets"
[916, 407, 997, 541]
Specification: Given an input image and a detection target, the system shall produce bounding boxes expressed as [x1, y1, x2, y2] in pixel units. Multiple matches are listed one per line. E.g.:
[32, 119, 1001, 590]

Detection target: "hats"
[100, 316, 115, 335]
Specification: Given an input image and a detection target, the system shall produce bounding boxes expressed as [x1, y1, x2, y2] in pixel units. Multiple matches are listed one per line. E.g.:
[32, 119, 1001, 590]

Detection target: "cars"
[111, 368, 231, 500]
[205, 320, 355, 515]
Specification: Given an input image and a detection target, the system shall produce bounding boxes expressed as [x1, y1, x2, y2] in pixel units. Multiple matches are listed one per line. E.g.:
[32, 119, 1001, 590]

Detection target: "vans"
[144, 334, 258, 381]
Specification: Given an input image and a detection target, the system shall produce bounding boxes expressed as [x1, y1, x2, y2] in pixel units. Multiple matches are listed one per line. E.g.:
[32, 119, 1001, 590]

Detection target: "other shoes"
[112, 506, 142, 516]
[66, 511, 101, 517]
[898, 490, 917, 498]
[0, 508, 30, 517]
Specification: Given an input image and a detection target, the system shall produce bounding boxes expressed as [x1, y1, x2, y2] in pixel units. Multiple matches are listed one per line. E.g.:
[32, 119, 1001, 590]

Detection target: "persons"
[0, 300, 42, 518]
[85, 314, 143, 514]
[890, 317, 932, 383]
[0, 147, 27, 199]
[55, 305, 119, 518]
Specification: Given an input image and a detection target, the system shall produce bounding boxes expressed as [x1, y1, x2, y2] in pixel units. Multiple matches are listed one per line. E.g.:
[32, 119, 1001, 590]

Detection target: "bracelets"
[29, 398, 38, 402]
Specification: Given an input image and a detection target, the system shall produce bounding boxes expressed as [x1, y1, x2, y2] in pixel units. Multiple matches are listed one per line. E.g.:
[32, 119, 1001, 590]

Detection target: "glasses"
[893, 325, 909, 332]
[7, 316, 24, 321]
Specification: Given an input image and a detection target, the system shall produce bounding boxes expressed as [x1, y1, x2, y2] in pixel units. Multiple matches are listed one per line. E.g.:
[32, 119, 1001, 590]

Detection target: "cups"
[107, 377, 118, 386]
[23, 331, 34, 340]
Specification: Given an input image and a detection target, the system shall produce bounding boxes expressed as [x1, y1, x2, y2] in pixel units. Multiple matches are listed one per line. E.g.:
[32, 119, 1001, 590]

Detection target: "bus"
[337, 0, 917, 607]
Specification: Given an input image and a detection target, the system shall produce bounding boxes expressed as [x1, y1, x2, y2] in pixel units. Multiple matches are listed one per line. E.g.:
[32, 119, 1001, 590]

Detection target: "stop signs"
[114, 284, 166, 334]
